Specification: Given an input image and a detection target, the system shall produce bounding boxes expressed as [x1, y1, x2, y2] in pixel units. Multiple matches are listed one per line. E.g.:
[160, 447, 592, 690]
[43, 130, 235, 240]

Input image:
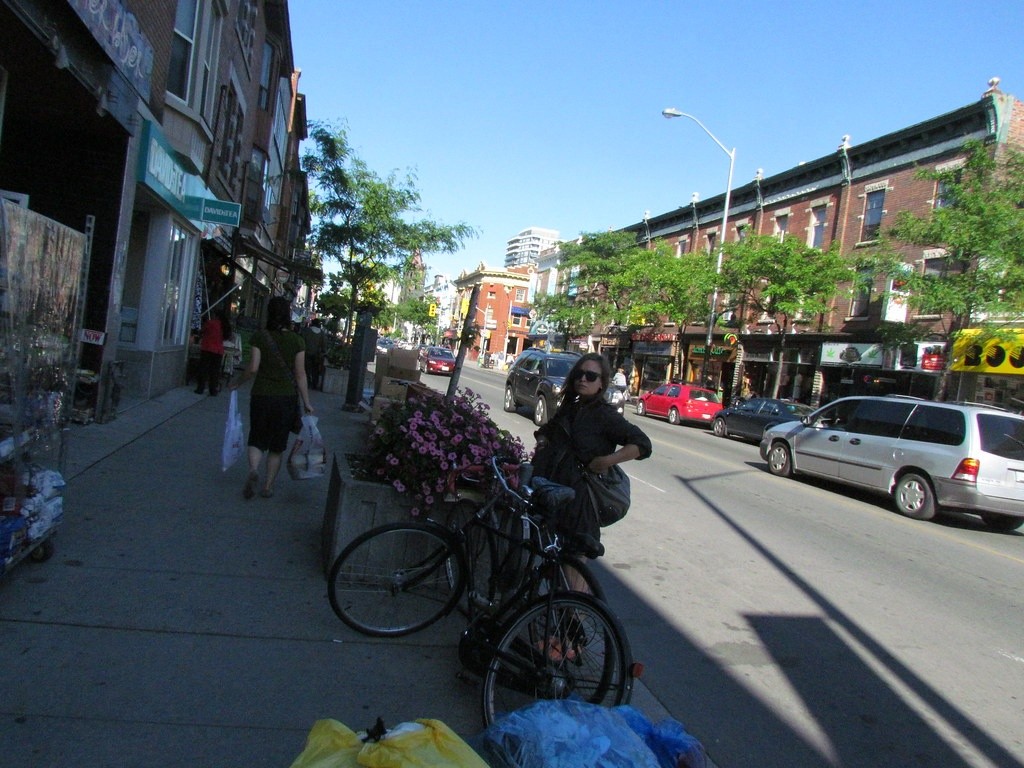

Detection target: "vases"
[326, 450, 526, 589]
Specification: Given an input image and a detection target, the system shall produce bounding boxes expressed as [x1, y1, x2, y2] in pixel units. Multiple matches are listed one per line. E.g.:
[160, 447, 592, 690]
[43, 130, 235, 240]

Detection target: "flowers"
[349, 375, 540, 521]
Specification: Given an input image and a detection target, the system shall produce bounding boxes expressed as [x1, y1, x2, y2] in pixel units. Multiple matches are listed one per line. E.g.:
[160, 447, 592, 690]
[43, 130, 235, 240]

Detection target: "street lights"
[662, 107, 736, 398]
[475, 302, 490, 356]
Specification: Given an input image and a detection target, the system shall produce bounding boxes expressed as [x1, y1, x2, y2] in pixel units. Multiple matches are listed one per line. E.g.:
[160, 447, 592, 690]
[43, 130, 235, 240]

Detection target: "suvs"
[504, 347, 625, 426]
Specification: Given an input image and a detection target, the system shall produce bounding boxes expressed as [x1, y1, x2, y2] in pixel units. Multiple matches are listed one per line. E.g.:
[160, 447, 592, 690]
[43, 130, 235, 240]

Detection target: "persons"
[527, 352, 652, 662]
[193, 307, 233, 397]
[243, 298, 314, 498]
[613, 368, 627, 390]
[291, 316, 326, 390]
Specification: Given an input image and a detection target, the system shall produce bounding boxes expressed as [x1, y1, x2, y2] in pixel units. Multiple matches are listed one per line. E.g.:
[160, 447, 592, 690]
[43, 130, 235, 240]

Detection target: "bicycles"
[327, 453, 643, 759]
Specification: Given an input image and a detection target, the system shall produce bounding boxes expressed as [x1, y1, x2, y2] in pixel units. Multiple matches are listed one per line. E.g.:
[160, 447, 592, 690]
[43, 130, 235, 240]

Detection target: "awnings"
[237, 236, 302, 294]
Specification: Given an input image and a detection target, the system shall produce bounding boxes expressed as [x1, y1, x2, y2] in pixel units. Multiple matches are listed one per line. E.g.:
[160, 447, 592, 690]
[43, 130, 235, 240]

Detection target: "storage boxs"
[368, 396, 405, 423]
[375, 374, 426, 398]
[375, 353, 421, 381]
[387, 348, 418, 369]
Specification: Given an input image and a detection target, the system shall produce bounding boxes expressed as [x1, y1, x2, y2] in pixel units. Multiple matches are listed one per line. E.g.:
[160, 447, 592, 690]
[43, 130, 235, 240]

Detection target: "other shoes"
[532, 635, 583, 661]
[208, 389, 219, 397]
[194, 386, 204, 395]
[242, 469, 259, 500]
[260, 479, 275, 498]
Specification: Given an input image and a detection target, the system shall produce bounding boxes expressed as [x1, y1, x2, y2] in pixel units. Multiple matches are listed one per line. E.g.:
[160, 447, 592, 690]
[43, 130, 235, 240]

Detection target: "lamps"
[272, 267, 289, 284]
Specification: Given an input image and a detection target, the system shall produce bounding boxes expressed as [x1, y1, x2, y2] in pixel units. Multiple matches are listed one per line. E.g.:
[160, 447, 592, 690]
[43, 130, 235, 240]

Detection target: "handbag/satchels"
[288, 414, 328, 480]
[221, 389, 245, 473]
[581, 461, 631, 527]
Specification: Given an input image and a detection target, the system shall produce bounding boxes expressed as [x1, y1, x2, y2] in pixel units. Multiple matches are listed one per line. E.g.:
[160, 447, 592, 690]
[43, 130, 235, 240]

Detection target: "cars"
[375, 338, 428, 359]
[636, 384, 725, 430]
[710, 397, 829, 447]
[420, 347, 457, 377]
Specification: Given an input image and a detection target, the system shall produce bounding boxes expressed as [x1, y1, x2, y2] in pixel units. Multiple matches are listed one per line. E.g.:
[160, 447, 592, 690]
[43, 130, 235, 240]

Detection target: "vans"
[759, 394, 1024, 531]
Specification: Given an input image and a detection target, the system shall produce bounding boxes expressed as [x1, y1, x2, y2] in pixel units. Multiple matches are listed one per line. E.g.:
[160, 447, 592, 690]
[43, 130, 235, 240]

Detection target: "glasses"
[573, 367, 603, 383]
